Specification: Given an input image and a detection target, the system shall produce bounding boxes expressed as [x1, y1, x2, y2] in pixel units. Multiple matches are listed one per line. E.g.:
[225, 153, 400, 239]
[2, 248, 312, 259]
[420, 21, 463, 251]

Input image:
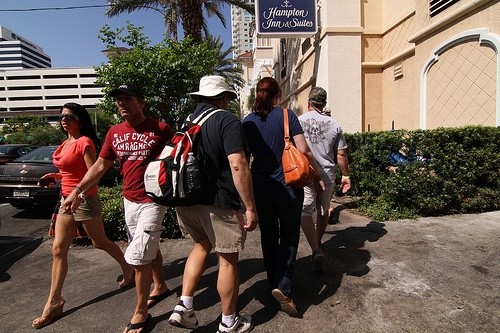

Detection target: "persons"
[31, 103, 135, 329]
[241, 77, 326, 315]
[297, 87, 351, 273]
[61, 84, 174, 333]
[167, 75, 255, 333]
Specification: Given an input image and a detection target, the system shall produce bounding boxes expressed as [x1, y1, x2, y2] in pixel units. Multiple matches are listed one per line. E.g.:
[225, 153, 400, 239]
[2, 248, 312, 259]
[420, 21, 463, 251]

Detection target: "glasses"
[59, 114, 78, 120]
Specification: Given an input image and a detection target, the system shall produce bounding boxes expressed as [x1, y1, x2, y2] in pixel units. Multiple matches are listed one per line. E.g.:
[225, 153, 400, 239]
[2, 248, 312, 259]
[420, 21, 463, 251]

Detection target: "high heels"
[119, 271, 135, 288]
[32, 302, 66, 329]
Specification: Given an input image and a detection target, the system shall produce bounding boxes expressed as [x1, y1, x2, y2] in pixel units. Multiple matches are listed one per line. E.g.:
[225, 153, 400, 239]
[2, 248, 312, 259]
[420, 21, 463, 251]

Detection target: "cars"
[0, 145, 122, 209]
[0, 144, 37, 166]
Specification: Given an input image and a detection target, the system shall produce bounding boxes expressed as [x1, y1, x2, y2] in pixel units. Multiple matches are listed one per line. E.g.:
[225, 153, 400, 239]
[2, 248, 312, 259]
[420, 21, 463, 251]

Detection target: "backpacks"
[144, 106, 227, 207]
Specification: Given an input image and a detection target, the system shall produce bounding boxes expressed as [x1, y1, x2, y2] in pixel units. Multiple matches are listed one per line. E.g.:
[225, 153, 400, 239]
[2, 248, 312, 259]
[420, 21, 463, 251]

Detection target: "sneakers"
[167, 300, 199, 330]
[217, 314, 255, 333]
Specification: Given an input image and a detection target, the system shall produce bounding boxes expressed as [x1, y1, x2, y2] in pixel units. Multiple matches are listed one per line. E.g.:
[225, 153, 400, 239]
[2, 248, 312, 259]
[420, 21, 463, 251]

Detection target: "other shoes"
[271, 289, 299, 317]
[311, 244, 329, 272]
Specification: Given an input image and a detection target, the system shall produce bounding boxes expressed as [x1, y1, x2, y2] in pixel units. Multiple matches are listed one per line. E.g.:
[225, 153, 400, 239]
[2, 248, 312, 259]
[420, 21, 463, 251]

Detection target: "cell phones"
[39, 176, 57, 187]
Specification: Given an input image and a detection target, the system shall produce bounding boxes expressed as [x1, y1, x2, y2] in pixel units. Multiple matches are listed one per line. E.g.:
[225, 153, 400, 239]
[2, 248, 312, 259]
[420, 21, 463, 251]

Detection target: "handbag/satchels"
[48, 201, 88, 239]
[282, 108, 317, 189]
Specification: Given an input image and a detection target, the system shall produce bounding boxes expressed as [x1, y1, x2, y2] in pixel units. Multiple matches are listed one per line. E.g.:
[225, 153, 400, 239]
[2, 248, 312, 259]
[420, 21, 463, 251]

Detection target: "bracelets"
[76, 186, 82, 192]
[342, 175, 349, 179]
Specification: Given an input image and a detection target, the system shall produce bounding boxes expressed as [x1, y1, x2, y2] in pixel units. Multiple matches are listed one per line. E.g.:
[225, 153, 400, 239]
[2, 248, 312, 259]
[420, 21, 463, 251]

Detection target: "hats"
[309, 88, 327, 104]
[189, 76, 237, 102]
[109, 83, 144, 99]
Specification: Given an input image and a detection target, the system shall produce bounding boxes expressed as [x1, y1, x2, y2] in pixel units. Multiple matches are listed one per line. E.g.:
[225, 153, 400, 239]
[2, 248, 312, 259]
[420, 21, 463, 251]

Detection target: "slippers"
[147, 288, 171, 309]
[122, 313, 152, 333]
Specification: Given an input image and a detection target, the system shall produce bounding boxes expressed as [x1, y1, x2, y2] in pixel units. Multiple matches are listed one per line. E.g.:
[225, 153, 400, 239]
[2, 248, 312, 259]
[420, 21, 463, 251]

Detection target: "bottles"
[186, 152, 200, 191]
